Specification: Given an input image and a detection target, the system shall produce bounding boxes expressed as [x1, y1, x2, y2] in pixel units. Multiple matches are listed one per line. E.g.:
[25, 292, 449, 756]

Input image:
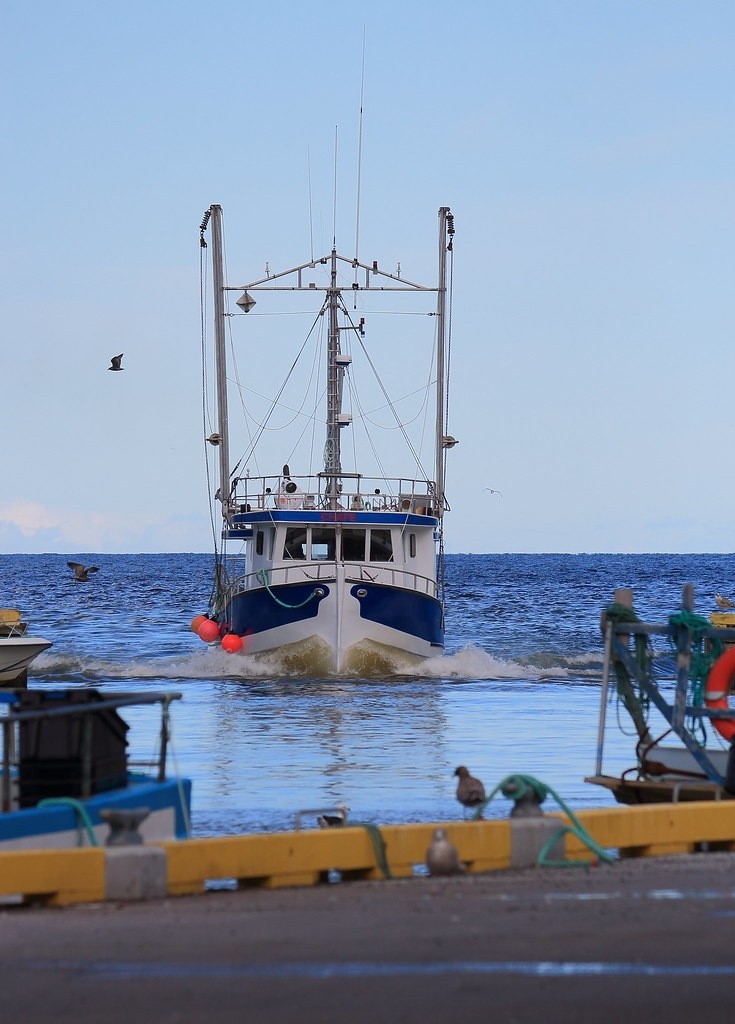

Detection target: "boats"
[191, 21, 461, 682]
[583, 582, 734, 808]
[0, 609, 193, 850]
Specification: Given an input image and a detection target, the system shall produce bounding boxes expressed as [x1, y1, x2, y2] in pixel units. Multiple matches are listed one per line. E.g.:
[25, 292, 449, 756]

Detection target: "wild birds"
[107, 353, 124, 371]
[481, 487, 502, 498]
[317, 804, 351, 830]
[452, 765, 488, 821]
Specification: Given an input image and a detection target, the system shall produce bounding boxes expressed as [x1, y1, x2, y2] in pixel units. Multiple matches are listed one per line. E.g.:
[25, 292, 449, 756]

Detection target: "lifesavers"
[706, 642, 735, 741]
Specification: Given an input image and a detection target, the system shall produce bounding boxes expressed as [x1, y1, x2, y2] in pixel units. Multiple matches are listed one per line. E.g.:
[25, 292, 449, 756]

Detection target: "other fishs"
[67, 562, 100, 582]
